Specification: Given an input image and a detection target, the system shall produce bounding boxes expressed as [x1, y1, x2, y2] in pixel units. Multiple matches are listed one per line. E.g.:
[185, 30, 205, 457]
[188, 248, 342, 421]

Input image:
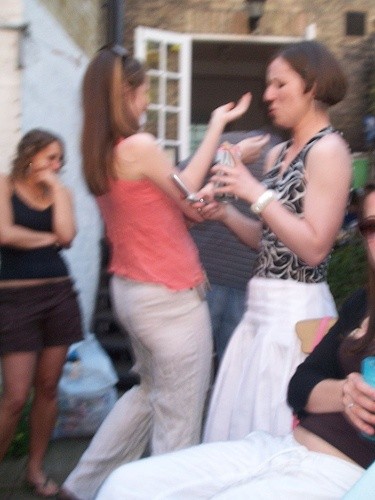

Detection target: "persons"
[193, 84, 287, 392]
[57, 45, 273, 500]
[0, 129, 86, 496]
[179, 39, 351, 444]
[92, 177, 375, 500]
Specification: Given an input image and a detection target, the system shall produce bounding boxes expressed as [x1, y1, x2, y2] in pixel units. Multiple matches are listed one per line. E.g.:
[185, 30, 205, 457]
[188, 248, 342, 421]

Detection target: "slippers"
[27, 473, 59, 496]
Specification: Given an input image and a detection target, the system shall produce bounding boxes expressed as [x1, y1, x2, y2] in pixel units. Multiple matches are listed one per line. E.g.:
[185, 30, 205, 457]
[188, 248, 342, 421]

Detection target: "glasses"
[354, 215, 375, 240]
[98, 41, 128, 57]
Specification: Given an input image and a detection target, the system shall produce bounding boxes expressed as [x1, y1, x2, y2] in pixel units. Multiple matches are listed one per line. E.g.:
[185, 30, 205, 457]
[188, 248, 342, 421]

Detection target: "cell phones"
[171, 175, 209, 206]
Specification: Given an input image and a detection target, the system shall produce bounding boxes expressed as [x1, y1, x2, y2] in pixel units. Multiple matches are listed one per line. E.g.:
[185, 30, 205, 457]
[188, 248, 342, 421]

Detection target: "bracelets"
[250, 189, 279, 218]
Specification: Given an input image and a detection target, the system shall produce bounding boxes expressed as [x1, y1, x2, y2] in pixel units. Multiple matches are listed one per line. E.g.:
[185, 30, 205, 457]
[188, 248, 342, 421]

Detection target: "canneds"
[213, 143, 242, 202]
